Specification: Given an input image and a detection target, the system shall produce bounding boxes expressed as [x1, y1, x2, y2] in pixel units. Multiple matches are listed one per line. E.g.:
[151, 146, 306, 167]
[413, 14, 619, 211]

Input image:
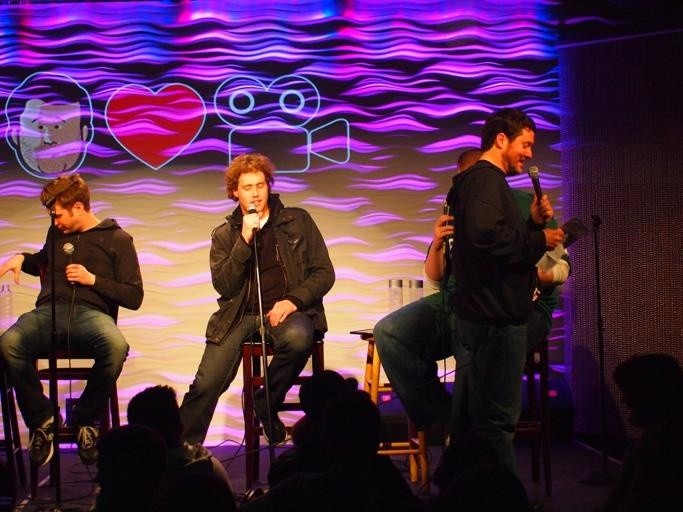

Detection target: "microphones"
[527, 165, 542, 206]
[246, 202, 256, 231]
[61, 243, 75, 285]
[443, 202, 451, 242]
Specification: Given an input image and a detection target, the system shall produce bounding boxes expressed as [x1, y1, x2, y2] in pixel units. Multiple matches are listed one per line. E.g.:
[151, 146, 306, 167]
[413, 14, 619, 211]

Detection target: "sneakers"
[28, 415, 54, 468]
[253, 404, 286, 444]
[76, 422, 99, 464]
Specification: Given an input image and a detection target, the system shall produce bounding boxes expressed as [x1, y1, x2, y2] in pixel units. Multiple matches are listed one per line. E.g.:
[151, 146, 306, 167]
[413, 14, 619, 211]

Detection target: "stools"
[242, 341, 325, 489]
[350, 329, 428, 487]
[30, 349, 120, 501]
[0, 387, 28, 496]
[514, 337, 552, 496]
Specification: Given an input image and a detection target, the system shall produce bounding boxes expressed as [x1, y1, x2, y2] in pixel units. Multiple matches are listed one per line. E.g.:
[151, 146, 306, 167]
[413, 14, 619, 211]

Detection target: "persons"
[441, 107, 563, 478]
[0, 171, 144, 466]
[371, 149, 573, 447]
[180, 151, 334, 446]
[86, 347, 682, 511]
